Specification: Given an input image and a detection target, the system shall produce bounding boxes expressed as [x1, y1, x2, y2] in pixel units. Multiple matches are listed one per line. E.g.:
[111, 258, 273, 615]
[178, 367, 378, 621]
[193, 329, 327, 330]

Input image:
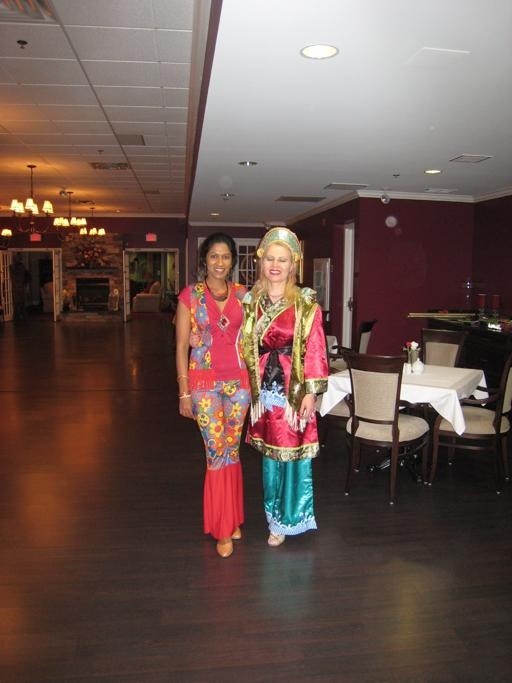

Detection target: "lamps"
[0, 165, 106, 243]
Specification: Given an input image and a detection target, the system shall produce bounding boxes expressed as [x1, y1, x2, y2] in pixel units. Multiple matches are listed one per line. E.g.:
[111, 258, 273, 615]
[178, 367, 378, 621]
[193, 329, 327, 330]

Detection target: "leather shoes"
[231, 528, 241, 540]
[268, 532, 285, 546]
[216, 537, 233, 557]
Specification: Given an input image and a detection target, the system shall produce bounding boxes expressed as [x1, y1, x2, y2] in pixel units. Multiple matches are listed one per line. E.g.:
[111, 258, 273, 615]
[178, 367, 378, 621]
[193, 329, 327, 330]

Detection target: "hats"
[257, 227, 301, 262]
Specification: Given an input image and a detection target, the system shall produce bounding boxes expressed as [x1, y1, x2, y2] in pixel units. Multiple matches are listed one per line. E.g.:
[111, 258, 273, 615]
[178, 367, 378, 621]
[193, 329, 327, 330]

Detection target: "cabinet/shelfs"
[427, 309, 512, 418]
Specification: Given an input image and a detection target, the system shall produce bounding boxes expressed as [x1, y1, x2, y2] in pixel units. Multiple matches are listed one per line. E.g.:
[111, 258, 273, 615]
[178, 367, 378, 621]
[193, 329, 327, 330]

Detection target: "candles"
[478, 294, 501, 309]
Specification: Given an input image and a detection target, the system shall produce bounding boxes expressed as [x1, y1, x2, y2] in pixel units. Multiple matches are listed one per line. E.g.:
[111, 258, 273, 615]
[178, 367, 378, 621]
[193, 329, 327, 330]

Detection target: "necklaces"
[205, 276, 228, 302]
[270, 295, 283, 299]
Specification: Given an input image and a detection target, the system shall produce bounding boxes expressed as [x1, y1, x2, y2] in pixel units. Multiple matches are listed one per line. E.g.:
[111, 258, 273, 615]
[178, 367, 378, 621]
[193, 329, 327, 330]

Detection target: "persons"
[189, 226, 329, 548]
[175, 233, 252, 558]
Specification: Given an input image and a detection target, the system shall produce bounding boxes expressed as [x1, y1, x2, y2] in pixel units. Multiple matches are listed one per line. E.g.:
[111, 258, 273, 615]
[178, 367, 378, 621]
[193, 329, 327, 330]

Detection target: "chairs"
[133, 280, 161, 313]
[317, 320, 512, 505]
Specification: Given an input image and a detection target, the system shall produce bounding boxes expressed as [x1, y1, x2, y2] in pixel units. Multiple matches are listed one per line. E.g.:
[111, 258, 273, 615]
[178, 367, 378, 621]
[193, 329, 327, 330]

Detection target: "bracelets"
[314, 393, 318, 401]
[176, 375, 189, 382]
[178, 392, 191, 399]
